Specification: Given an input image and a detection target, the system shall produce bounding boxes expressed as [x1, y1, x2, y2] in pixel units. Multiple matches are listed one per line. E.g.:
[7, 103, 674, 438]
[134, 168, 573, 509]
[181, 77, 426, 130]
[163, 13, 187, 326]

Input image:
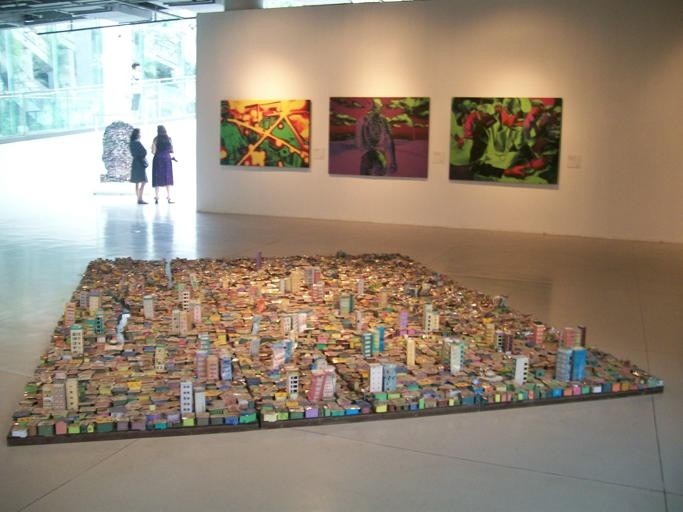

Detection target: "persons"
[129, 62, 140, 113]
[130, 128, 149, 205]
[151, 125, 177, 203]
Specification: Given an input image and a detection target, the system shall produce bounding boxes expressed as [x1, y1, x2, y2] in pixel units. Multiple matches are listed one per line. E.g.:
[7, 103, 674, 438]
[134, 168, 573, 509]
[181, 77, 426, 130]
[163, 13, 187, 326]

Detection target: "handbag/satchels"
[140, 158, 147, 167]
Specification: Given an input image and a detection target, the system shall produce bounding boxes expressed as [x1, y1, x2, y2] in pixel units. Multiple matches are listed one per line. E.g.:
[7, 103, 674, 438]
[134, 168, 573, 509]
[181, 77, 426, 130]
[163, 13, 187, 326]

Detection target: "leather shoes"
[137, 200, 148, 204]
[154, 197, 174, 203]
